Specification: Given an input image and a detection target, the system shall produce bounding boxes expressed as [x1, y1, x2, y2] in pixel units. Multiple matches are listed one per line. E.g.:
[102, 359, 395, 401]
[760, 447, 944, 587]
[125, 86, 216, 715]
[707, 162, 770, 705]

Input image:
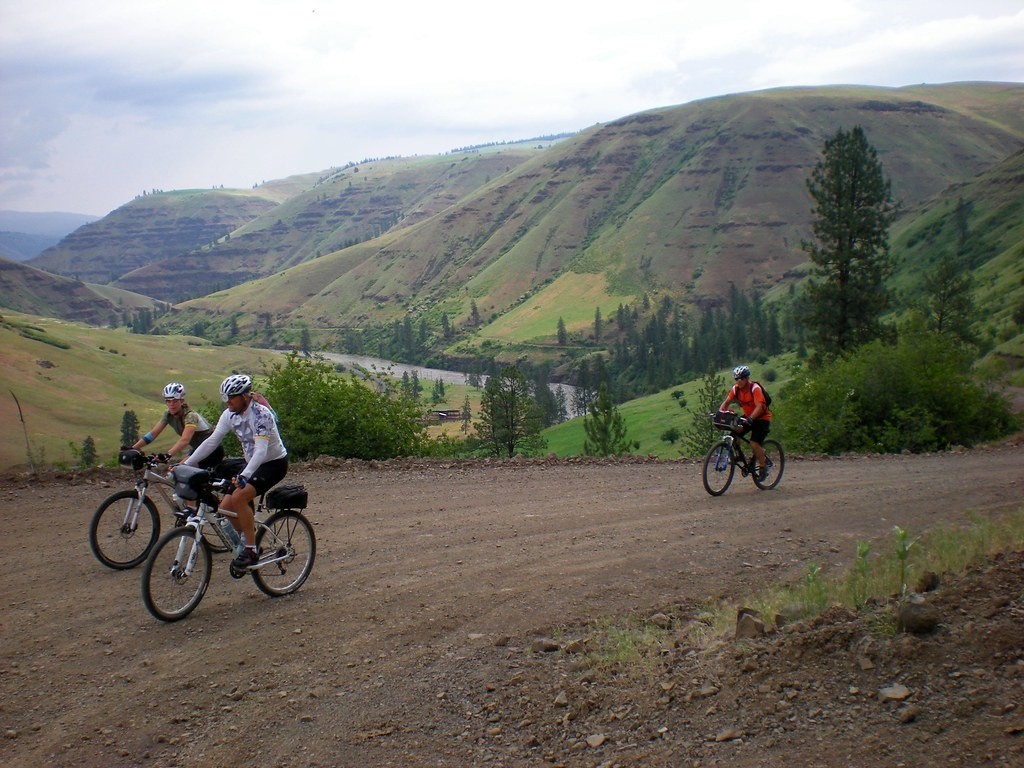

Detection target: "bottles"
[236, 534, 246, 554]
[221, 519, 241, 547]
[172, 493, 186, 512]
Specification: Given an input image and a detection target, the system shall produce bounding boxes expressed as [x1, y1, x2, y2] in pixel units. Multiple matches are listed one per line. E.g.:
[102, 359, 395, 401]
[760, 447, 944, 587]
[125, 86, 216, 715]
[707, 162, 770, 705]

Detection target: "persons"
[120, 382, 226, 515]
[719, 366, 771, 483]
[167, 375, 288, 569]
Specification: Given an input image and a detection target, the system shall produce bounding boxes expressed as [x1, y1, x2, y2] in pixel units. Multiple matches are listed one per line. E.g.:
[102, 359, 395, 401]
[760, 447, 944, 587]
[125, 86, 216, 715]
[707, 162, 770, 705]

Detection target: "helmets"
[732, 365, 750, 378]
[220, 375, 251, 396]
[162, 383, 184, 400]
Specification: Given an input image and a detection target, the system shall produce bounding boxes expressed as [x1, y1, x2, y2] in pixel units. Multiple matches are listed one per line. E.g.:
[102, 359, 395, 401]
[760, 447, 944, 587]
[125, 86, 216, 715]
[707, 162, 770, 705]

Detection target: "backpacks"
[736, 381, 771, 407]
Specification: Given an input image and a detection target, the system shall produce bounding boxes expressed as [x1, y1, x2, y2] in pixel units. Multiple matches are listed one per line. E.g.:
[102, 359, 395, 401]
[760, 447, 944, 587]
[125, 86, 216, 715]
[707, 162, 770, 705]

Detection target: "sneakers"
[233, 547, 259, 569]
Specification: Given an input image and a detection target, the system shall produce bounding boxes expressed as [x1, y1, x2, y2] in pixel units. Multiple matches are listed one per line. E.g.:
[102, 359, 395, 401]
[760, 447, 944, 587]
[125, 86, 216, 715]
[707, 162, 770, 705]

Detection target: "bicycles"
[143, 463, 317, 624]
[90, 448, 256, 569]
[702, 412, 784, 496]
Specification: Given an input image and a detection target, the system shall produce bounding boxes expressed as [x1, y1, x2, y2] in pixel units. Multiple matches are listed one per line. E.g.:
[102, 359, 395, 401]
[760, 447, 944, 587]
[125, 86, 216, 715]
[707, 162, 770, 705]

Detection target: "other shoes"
[728, 454, 741, 464]
[757, 463, 768, 482]
[181, 507, 193, 518]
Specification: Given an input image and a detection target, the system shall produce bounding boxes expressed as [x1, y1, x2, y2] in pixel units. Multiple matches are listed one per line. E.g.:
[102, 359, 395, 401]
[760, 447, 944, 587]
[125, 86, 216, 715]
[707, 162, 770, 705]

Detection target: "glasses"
[735, 378, 743, 381]
